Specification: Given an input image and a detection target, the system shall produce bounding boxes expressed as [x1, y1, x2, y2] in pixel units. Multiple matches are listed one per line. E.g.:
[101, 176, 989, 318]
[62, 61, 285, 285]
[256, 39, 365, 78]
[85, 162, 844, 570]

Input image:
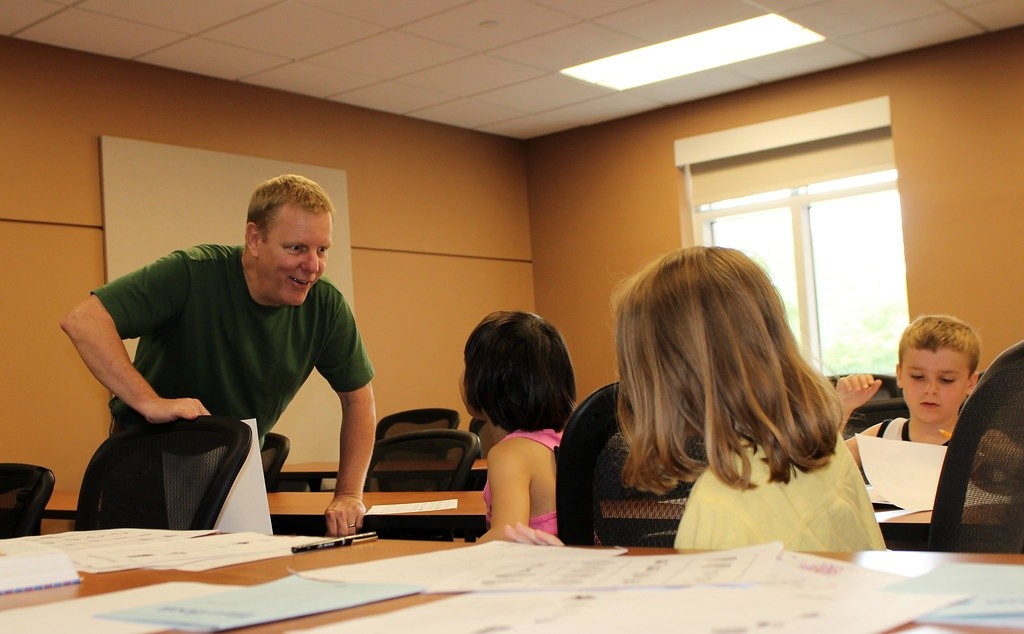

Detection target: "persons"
[458, 310, 578, 545]
[833, 312, 1024, 487]
[59, 172, 376, 540]
[503, 245, 890, 551]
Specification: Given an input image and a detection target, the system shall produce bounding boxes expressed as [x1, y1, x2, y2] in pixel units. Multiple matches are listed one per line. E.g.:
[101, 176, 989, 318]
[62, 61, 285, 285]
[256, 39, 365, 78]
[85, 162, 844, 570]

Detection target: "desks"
[0, 458, 1024, 634]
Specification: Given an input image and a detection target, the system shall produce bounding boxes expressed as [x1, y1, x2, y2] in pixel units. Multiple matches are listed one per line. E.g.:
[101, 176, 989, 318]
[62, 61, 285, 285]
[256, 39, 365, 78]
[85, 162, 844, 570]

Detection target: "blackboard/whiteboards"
[98, 134, 356, 331]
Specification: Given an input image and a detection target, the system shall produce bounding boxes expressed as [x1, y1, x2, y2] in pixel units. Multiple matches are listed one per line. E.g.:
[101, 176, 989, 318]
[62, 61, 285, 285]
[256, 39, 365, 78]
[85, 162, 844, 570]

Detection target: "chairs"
[827, 374, 910, 446]
[364, 408, 486, 492]
[555, 382, 710, 547]
[0, 462, 55, 539]
[928, 341, 1024, 555]
[260, 433, 291, 493]
[75, 416, 253, 532]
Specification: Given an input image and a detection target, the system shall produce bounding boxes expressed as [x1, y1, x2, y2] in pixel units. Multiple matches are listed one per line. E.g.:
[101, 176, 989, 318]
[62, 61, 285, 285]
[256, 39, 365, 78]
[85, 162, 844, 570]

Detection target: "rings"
[348, 523, 354, 528]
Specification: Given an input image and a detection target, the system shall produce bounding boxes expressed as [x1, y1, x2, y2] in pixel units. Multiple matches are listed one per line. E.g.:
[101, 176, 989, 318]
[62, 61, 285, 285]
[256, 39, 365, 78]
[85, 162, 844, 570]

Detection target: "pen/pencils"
[938, 428, 982, 455]
[292, 531, 378, 553]
